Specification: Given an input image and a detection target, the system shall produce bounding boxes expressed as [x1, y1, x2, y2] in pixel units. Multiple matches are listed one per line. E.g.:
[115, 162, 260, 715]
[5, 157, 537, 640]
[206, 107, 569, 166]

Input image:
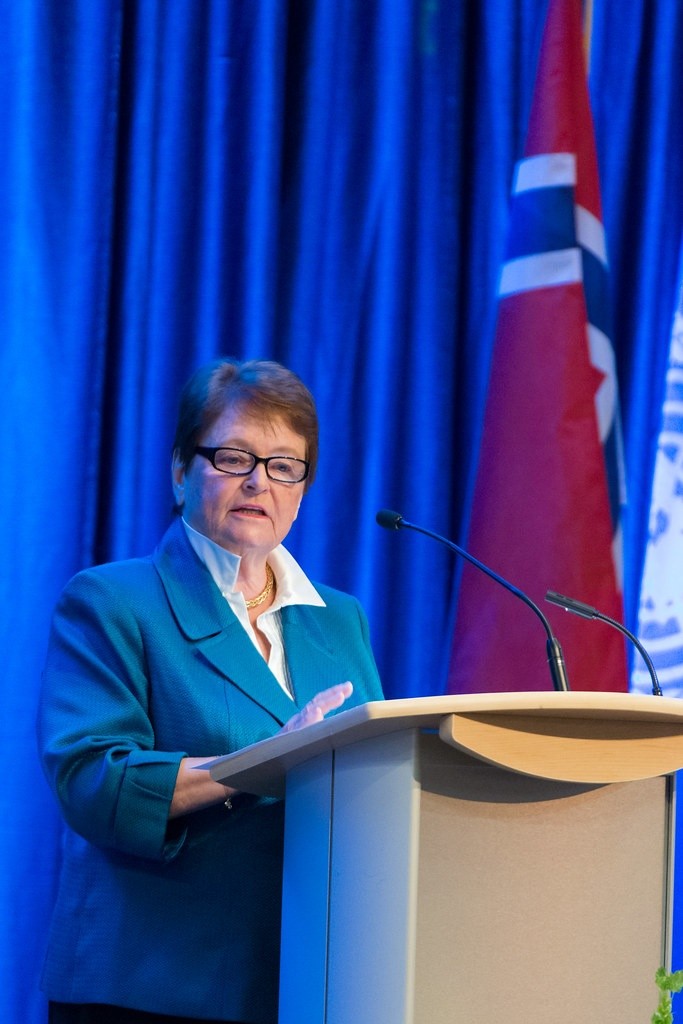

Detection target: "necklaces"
[222, 785, 232, 809]
[247, 563, 274, 609]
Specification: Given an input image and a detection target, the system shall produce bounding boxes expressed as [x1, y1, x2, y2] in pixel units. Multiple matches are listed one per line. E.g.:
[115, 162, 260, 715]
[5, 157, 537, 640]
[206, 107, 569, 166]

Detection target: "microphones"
[545, 589, 662, 696]
[376, 509, 570, 691]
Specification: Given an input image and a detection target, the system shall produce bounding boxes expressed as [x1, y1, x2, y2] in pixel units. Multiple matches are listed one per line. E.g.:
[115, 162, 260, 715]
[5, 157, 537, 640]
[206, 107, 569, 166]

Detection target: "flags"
[446, 1, 638, 691]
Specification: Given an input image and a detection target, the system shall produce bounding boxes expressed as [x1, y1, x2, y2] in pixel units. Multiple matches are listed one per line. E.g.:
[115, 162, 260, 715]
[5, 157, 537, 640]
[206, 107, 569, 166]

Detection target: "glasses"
[195, 446, 311, 484]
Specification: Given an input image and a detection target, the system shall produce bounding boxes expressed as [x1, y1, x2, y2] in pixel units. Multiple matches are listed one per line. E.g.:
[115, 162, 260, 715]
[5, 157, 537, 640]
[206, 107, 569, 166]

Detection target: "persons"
[33, 359, 383, 1024]
